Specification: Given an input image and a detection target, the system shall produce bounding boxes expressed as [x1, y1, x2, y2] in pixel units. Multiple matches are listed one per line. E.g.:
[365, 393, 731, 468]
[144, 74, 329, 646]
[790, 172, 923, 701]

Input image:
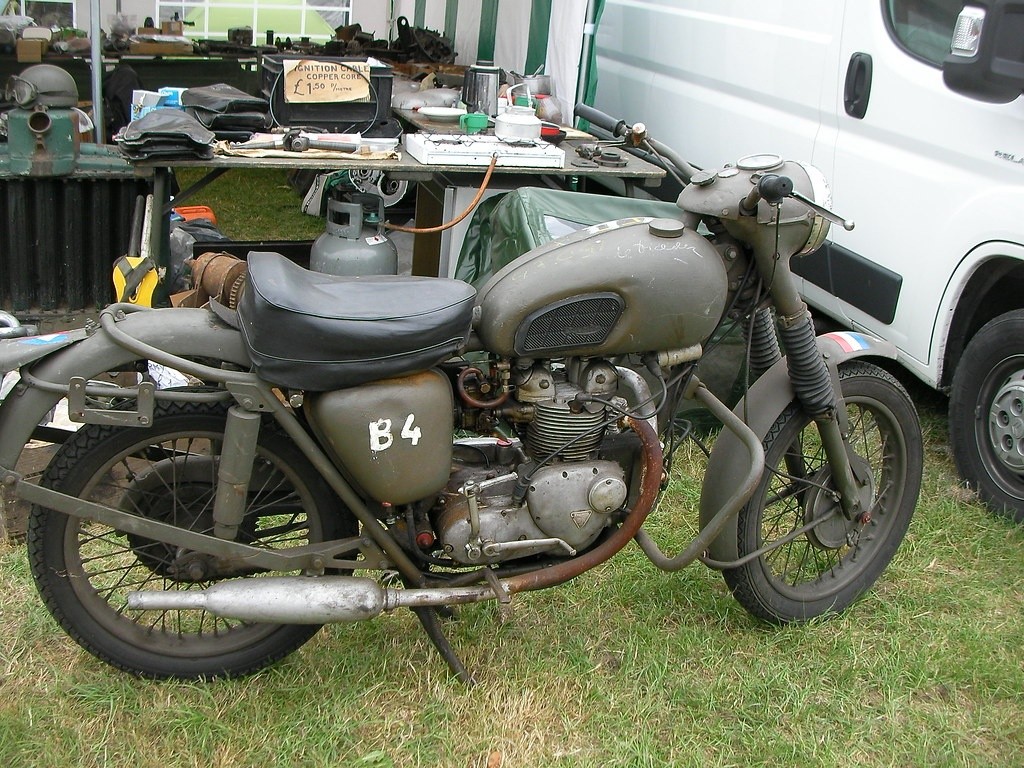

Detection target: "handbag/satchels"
[287, 168, 334, 200]
[301, 169, 349, 217]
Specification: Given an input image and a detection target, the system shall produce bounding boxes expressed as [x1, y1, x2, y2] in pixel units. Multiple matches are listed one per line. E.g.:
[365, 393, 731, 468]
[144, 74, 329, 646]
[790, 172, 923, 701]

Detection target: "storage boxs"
[130, 40, 194, 56]
[158, 87, 188, 106]
[16, 38, 43, 63]
[130, 105, 181, 127]
[407, 133, 565, 170]
[261, 54, 395, 131]
[162, 22, 184, 37]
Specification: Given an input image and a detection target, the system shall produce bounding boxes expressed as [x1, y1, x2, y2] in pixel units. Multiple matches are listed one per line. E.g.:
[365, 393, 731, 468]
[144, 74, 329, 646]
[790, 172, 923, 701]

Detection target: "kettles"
[462, 60, 501, 116]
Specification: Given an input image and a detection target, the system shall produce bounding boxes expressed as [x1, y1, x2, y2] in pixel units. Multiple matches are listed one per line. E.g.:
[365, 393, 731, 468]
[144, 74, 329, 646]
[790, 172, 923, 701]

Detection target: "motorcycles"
[0, 101, 926, 690]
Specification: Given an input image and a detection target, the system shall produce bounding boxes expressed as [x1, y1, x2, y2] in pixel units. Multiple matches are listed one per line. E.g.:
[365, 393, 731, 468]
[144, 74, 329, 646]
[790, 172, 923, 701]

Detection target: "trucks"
[392, 0, 1024, 526]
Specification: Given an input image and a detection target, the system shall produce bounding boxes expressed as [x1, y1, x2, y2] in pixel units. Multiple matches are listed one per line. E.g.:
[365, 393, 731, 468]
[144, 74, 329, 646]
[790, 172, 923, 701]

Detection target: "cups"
[459, 113, 488, 135]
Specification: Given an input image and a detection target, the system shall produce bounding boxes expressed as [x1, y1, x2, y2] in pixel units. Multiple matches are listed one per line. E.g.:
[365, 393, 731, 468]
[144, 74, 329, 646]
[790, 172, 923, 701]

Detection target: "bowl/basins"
[514, 74, 551, 95]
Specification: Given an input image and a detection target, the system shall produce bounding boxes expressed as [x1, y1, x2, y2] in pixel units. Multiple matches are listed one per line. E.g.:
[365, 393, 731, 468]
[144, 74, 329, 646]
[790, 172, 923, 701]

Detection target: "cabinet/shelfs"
[410, 173, 518, 280]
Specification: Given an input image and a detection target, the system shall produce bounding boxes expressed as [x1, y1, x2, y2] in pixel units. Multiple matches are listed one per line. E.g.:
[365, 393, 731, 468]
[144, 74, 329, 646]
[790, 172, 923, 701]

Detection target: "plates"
[416, 107, 465, 122]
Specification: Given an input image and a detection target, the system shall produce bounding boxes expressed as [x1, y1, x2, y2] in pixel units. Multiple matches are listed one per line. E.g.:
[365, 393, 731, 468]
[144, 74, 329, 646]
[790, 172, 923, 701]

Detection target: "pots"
[492, 113, 567, 143]
